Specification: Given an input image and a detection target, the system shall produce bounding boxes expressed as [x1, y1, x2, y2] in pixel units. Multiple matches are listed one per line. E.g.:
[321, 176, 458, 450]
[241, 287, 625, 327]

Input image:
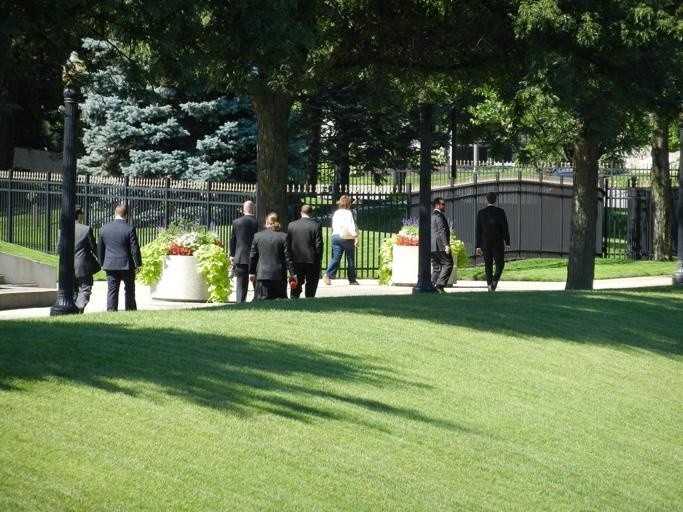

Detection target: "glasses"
[442, 204, 446, 206]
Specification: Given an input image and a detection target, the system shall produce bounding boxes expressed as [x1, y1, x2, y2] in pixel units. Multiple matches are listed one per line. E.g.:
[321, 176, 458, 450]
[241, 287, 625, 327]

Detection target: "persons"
[287, 203, 323, 299]
[96, 204, 143, 310]
[249, 211, 299, 297]
[54, 203, 97, 313]
[229, 199, 259, 303]
[322, 195, 361, 285]
[474, 192, 511, 292]
[430, 197, 454, 293]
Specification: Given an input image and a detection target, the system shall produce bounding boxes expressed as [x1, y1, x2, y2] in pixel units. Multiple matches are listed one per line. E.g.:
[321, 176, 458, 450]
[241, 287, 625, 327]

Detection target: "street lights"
[412, 90, 436, 293]
[50, 52, 87, 316]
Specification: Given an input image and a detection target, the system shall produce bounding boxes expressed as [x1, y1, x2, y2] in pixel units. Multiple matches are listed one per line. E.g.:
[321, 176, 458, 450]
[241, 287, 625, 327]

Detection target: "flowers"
[379, 216, 469, 285]
[136, 217, 231, 302]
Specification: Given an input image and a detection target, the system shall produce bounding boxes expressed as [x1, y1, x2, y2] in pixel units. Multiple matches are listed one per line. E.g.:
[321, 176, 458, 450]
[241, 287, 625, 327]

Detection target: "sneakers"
[349, 280, 360, 285]
[322, 274, 331, 285]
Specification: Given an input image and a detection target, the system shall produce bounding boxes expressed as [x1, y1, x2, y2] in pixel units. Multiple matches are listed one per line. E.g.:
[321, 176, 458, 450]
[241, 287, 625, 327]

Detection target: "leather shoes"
[434, 283, 445, 293]
[489, 284, 495, 292]
